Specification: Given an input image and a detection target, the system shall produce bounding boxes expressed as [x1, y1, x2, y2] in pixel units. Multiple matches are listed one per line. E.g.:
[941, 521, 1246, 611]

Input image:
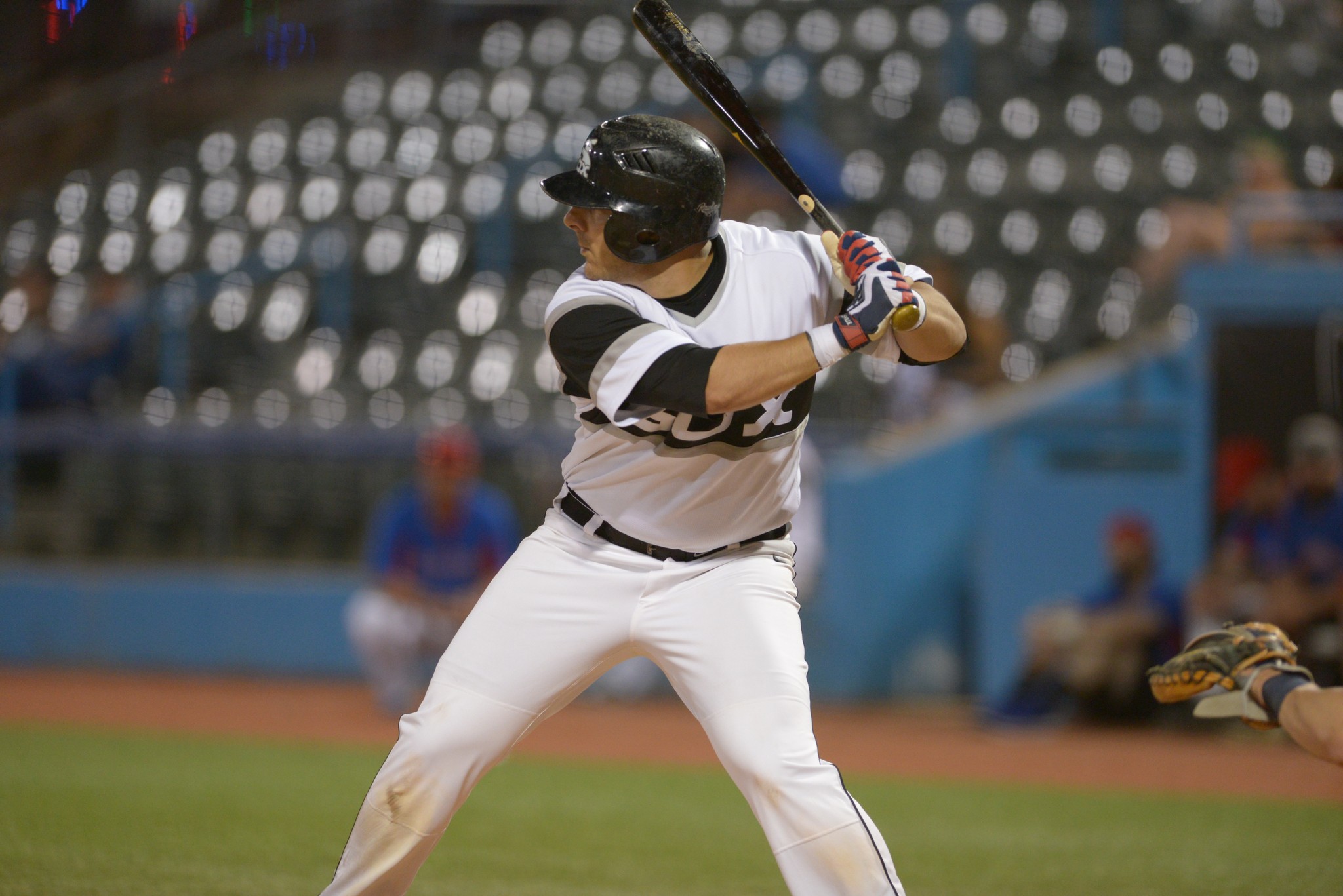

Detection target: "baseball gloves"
[1149, 620, 1313, 732]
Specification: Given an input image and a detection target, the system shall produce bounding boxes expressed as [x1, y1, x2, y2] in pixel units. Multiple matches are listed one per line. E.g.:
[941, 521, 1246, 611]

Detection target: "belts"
[559, 487, 790, 562]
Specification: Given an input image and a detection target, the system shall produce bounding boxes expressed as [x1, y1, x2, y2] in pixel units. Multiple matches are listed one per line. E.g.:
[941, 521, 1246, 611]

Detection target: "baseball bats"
[630, 1, 921, 330]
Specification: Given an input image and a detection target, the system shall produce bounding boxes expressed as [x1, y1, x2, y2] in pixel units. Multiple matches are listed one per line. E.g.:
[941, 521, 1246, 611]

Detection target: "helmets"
[539, 112, 724, 261]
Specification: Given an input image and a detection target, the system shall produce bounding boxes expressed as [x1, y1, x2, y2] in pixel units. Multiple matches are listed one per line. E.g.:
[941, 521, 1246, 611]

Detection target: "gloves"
[821, 229, 933, 299]
[804, 259, 926, 372]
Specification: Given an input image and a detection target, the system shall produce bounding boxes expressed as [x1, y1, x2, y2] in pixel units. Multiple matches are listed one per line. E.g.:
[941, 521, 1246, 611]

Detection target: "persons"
[972, 412, 1343, 773]
[338, 418, 523, 737]
[307, 100, 969, 894]
